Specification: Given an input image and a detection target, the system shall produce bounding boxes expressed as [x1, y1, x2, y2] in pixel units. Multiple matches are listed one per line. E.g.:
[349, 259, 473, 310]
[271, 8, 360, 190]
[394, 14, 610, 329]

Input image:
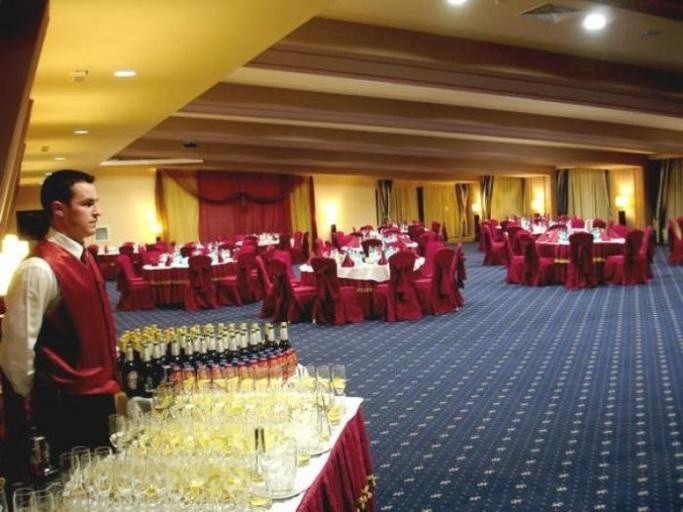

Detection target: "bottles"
[115, 322, 298, 398]
[34, 437, 62, 487]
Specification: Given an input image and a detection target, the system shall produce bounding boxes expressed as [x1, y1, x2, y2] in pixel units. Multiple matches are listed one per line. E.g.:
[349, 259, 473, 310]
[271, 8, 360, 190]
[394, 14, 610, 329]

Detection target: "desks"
[95, 255, 121, 280]
[63, 396, 377, 512]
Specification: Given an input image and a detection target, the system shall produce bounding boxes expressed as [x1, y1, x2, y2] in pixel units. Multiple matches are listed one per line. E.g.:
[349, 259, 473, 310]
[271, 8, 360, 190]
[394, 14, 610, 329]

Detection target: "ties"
[80, 249, 87, 265]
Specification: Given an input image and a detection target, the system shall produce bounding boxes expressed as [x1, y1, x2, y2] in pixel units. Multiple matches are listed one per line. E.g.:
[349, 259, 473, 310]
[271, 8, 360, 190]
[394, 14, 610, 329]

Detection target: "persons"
[0, 169, 121, 475]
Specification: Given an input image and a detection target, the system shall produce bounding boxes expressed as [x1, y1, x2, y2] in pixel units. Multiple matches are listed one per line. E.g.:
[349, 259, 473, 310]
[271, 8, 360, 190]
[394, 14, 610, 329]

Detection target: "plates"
[311, 439, 329, 455]
[253, 482, 307, 499]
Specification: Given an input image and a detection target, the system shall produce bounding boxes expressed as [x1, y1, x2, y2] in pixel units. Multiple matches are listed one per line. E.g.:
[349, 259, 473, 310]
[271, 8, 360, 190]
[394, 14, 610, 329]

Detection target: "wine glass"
[0, 480, 271, 512]
[61, 434, 310, 481]
[110, 363, 346, 433]
